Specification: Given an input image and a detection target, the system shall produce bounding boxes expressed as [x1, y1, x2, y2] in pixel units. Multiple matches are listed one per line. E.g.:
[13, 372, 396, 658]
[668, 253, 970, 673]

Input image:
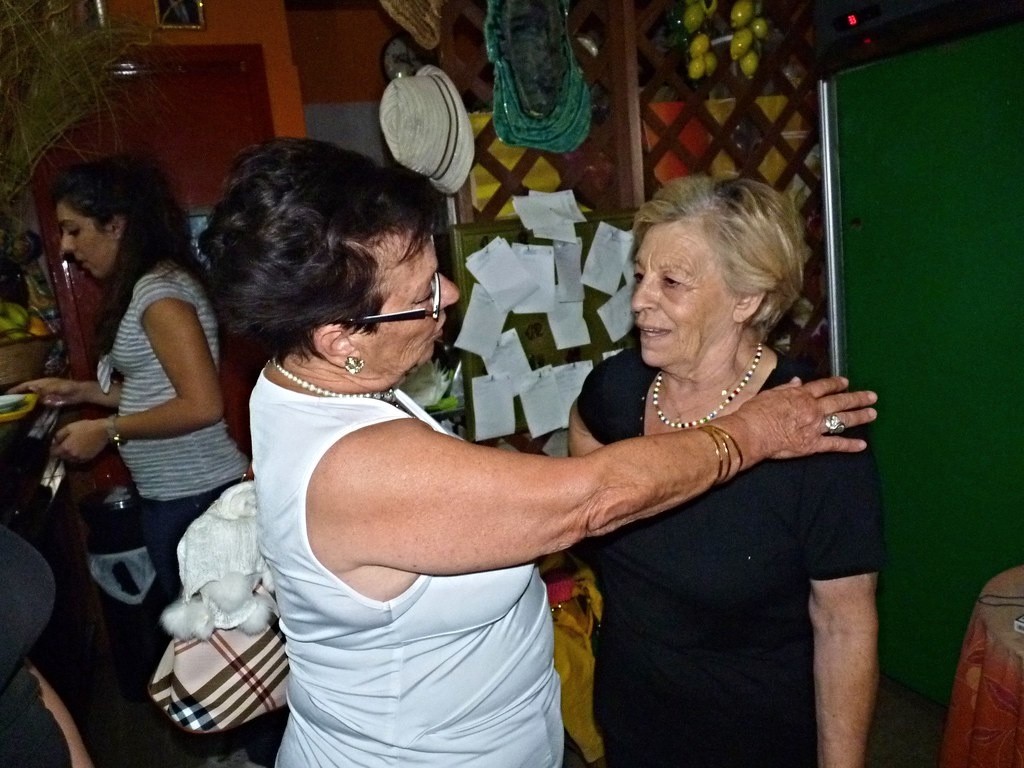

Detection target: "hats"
[379, 65, 477, 196]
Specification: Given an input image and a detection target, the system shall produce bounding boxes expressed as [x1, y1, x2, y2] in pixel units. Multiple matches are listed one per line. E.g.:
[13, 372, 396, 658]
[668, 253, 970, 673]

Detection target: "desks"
[940, 564, 1024, 768]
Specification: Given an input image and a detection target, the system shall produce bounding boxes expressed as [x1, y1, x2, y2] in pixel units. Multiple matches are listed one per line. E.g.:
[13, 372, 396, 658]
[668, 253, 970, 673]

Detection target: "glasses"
[330, 269, 442, 325]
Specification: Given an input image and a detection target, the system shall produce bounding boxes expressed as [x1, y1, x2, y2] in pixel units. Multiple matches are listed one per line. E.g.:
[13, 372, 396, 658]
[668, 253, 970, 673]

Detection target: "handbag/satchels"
[145, 611, 291, 738]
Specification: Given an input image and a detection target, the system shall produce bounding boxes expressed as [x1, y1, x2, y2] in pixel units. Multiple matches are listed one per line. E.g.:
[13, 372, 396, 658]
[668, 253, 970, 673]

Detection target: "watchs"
[107, 415, 128, 447]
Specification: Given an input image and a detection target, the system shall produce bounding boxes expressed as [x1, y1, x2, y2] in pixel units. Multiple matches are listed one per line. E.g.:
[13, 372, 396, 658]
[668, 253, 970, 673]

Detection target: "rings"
[825, 415, 845, 434]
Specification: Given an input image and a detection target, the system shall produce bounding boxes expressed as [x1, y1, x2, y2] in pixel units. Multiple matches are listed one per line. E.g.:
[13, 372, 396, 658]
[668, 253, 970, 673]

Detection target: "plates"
[0, 393, 39, 422]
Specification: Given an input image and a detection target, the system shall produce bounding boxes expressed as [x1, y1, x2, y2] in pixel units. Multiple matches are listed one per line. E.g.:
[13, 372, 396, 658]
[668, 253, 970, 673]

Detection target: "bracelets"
[697, 425, 744, 485]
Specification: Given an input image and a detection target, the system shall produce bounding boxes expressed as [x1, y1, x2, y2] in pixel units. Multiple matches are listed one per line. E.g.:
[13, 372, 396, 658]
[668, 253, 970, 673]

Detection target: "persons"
[6, 155, 285, 768]
[196, 136, 878, 768]
[0, 524, 96, 768]
[570, 175, 887, 768]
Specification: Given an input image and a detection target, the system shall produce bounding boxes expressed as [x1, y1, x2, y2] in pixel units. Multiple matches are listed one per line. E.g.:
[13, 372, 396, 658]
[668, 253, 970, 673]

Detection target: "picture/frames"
[153, 0, 206, 30]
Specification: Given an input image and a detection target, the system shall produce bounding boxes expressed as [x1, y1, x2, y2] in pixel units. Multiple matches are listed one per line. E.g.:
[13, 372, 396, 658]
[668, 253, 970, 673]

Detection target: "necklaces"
[272, 358, 396, 403]
[652, 343, 763, 428]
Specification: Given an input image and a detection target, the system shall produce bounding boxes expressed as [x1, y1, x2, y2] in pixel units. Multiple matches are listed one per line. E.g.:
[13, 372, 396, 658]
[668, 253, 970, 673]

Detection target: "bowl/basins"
[0, 395, 26, 413]
